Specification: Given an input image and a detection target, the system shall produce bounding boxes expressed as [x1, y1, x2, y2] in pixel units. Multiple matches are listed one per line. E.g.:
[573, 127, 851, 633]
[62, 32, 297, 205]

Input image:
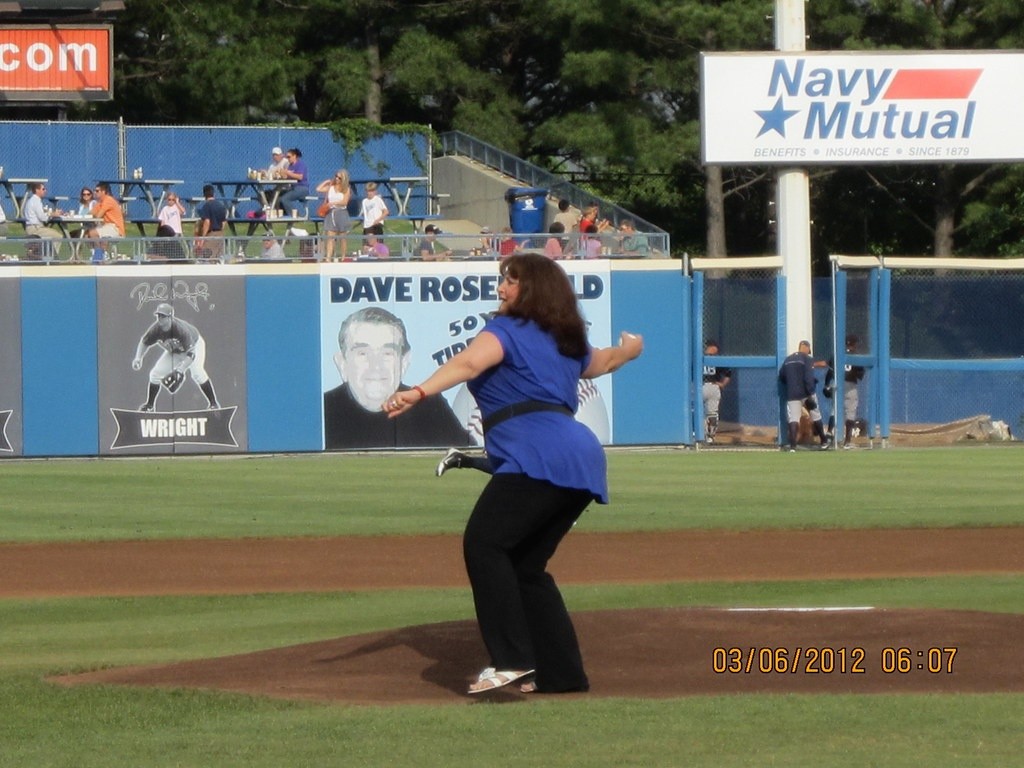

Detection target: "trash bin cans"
[508, 187, 548, 248]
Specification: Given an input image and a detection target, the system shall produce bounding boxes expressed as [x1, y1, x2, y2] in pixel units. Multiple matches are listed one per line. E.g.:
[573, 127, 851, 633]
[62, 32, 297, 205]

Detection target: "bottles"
[134, 167, 143, 179]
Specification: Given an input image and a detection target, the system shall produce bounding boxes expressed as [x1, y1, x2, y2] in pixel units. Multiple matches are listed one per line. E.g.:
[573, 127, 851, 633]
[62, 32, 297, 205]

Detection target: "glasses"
[335, 175, 342, 180]
[168, 199, 176, 201]
[96, 190, 102, 193]
[287, 155, 294, 159]
[83, 194, 91, 196]
[38, 188, 45, 191]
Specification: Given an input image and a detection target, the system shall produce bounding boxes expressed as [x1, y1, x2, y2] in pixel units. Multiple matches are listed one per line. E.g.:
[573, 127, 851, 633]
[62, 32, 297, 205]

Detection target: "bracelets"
[413, 386, 425, 401]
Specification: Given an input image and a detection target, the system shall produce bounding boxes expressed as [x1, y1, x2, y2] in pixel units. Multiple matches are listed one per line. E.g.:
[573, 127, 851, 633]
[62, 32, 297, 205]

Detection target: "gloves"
[805, 400, 818, 410]
[823, 386, 832, 397]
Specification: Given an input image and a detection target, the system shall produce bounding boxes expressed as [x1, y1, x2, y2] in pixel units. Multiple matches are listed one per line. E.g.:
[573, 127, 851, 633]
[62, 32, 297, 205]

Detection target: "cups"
[0, 254, 19, 262]
[45, 206, 75, 217]
[601, 246, 612, 254]
[247, 208, 298, 220]
[247, 167, 288, 180]
[89, 249, 130, 260]
[470, 248, 489, 256]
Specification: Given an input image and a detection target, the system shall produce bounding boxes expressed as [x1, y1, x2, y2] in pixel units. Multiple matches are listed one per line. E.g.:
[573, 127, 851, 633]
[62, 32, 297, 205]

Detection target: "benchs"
[0, 193, 451, 263]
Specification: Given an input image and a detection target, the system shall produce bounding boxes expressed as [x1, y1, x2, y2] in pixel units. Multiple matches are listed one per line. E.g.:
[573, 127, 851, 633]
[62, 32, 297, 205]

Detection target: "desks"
[131, 218, 199, 258]
[223, 218, 306, 259]
[0, 178, 48, 224]
[98, 179, 185, 224]
[349, 176, 428, 215]
[313, 214, 446, 255]
[14, 217, 106, 261]
[211, 179, 298, 218]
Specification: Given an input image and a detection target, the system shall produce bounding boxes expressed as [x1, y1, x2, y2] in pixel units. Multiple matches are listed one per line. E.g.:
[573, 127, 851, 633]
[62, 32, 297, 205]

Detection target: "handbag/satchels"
[317, 203, 329, 217]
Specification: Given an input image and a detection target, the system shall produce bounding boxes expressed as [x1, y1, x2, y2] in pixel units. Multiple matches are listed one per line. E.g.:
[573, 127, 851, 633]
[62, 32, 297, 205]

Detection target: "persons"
[24, 183, 63, 260]
[690, 342, 731, 443]
[67, 183, 126, 261]
[823, 335, 866, 449]
[324, 307, 478, 449]
[612, 219, 653, 259]
[479, 199, 609, 260]
[194, 185, 229, 258]
[779, 341, 832, 452]
[363, 230, 389, 257]
[418, 224, 452, 261]
[260, 232, 285, 258]
[436, 447, 495, 477]
[258, 147, 309, 229]
[199, 248, 212, 264]
[157, 192, 186, 234]
[315, 169, 351, 262]
[381, 252, 644, 694]
[357, 182, 389, 247]
[132, 303, 221, 412]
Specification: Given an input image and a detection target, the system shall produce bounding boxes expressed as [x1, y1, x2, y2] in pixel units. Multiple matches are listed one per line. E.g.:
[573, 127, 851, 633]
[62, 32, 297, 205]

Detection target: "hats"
[799, 341, 812, 355]
[846, 334, 859, 346]
[262, 232, 273, 240]
[425, 224, 441, 235]
[272, 147, 282, 155]
[480, 227, 492, 234]
[154, 303, 174, 316]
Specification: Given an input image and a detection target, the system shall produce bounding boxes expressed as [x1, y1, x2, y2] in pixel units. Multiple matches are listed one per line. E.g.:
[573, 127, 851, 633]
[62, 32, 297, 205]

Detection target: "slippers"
[520, 681, 536, 692]
[467, 667, 535, 693]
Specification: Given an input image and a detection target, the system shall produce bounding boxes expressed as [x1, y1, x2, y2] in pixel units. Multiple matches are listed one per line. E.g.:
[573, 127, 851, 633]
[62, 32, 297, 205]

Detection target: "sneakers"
[437, 447, 465, 477]
[821, 435, 832, 449]
[843, 443, 853, 450]
[790, 448, 796, 452]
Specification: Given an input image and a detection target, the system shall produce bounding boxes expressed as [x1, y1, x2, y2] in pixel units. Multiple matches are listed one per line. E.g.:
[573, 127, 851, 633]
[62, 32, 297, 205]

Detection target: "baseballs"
[619, 334, 636, 349]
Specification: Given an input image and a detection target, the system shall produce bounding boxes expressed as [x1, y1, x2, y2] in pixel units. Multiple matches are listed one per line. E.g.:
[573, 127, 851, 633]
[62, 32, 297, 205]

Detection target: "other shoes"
[140, 405, 154, 412]
[706, 437, 715, 444]
[209, 405, 219, 410]
[27, 249, 42, 260]
